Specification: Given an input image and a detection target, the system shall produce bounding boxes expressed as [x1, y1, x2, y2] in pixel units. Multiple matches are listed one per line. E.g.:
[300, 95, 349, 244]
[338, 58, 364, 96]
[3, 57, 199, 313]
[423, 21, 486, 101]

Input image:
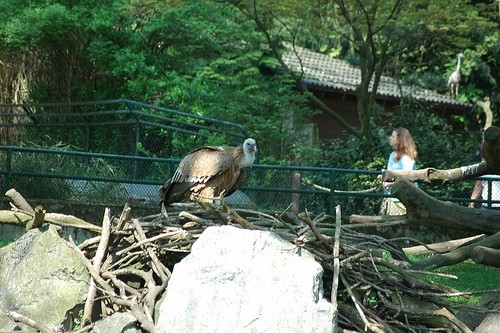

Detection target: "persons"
[378, 126, 418, 215]
[468, 126, 500, 210]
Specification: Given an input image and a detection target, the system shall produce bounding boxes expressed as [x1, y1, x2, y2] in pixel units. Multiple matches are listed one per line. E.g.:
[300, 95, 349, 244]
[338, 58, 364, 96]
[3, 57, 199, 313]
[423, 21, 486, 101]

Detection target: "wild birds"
[156, 138, 257, 208]
